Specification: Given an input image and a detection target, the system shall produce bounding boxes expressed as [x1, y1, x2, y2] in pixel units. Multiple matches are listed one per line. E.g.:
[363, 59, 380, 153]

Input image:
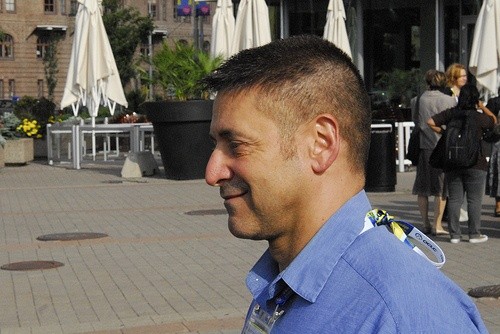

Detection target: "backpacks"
[442, 110, 485, 170]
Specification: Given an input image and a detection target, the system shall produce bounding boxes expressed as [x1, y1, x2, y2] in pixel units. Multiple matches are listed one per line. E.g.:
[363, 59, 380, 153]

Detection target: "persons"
[194, 34, 491, 334]
[406, 63, 500, 244]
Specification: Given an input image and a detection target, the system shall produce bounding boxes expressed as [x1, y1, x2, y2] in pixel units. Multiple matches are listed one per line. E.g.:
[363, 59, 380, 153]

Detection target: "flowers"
[17, 118, 43, 140]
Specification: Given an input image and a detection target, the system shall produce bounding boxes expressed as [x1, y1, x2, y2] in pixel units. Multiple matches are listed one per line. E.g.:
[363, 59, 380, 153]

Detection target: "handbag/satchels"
[406, 127, 422, 165]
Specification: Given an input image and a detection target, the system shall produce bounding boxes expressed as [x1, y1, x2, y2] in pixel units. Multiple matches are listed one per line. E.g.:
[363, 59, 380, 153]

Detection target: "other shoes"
[432, 228, 449, 235]
[423, 225, 430, 235]
[468, 233, 490, 243]
[459, 209, 469, 221]
[450, 238, 461, 244]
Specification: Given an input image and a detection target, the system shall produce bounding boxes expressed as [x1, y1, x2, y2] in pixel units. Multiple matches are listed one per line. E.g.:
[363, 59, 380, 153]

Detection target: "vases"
[5, 137, 34, 163]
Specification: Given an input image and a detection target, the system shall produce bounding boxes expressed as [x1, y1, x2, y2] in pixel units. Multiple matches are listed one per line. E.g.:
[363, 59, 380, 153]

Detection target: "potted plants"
[133, 38, 224, 181]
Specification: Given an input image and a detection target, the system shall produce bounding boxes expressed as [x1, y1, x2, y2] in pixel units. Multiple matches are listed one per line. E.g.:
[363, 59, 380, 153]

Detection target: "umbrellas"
[229, 0, 272, 53]
[208, 0, 236, 100]
[61, 0, 129, 159]
[469, 0, 500, 104]
[323, 0, 352, 61]
